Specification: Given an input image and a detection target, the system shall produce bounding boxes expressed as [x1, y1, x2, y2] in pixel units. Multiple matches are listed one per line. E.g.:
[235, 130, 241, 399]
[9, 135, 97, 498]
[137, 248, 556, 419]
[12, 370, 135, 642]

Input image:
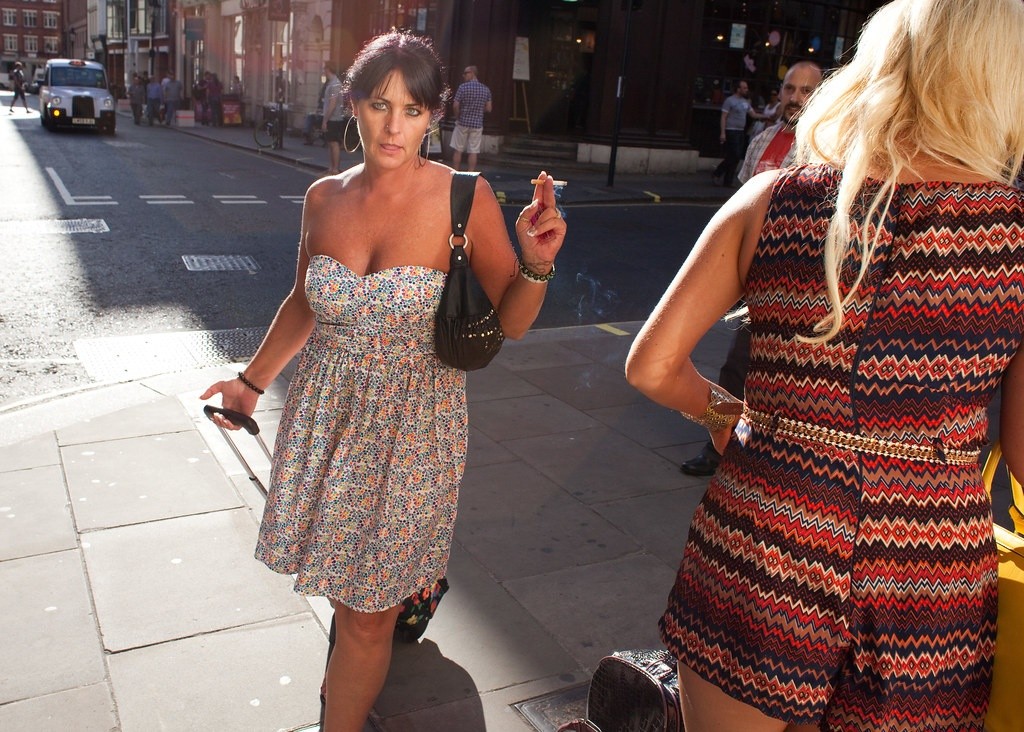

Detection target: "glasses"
[464, 71, 472, 75]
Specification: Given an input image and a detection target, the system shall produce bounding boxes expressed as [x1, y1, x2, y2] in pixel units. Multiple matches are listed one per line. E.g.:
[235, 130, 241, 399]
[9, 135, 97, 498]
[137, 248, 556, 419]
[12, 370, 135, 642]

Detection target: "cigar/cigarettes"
[531, 179, 568, 187]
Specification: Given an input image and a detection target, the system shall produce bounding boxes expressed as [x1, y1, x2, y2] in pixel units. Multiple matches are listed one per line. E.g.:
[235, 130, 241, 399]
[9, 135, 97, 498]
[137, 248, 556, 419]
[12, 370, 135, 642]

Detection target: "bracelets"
[675, 381, 736, 433]
[520, 257, 556, 281]
[237, 371, 264, 398]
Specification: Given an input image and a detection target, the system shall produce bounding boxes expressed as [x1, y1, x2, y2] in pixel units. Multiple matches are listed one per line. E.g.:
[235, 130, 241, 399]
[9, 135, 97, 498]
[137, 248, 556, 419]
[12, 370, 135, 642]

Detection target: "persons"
[198, 29, 569, 732]
[320, 60, 344, 176]
[623, 0, 1024, 731]
[714, 61, 821, 185]
[450, 66, 493, 172]
[9, 57, 248, 127]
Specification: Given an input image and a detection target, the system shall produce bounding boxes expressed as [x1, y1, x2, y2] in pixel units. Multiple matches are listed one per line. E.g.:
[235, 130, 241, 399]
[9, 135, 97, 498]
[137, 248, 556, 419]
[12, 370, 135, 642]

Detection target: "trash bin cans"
[263, 105, 287, 134]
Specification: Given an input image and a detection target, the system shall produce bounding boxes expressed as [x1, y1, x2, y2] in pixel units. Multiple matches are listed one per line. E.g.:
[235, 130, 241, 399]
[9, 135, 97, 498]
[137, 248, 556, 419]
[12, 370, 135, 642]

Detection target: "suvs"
[29, 69, 44, 91]
[37, 57, 116, 135]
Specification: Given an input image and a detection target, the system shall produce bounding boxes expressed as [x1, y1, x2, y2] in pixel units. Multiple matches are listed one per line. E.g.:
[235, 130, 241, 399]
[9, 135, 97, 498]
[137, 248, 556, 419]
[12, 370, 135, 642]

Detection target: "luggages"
[203, 405, 452, 640]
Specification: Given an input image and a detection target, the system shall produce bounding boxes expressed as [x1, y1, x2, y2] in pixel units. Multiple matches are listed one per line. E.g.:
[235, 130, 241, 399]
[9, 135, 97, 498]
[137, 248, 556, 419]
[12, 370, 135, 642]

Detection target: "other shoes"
[681, 442, 722, 476]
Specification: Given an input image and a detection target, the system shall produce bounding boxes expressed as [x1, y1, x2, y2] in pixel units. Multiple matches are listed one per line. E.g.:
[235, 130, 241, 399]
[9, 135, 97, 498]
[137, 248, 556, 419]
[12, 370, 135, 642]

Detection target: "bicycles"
[254, 106, 282, 147]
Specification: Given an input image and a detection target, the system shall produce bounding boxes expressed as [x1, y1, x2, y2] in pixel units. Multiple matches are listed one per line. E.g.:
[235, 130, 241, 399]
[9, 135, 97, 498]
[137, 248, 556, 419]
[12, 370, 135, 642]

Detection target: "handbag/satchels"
[437, 263, 506, 373]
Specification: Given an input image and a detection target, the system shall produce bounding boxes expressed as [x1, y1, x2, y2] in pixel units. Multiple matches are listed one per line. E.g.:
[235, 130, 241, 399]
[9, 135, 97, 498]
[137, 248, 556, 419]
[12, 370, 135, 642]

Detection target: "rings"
[518, 213, 532, 224]
[554, 207, 562, 220]
